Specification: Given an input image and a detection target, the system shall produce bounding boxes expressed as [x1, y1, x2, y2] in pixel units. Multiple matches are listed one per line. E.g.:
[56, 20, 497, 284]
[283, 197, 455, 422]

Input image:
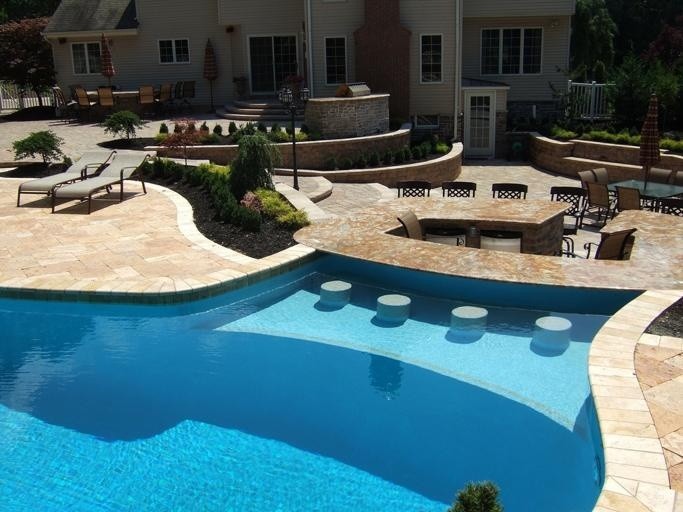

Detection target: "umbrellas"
[640, 93, 660, 192]
[203, 39, 220, 114]
[100, 34, 117, 88]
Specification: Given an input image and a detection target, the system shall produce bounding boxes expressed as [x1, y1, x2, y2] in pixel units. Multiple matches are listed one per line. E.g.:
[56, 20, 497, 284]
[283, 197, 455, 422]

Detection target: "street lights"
[278, 85, 310, 191]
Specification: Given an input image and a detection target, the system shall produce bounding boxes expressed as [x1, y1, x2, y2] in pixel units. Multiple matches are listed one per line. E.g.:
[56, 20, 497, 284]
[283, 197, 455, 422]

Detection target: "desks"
[604, 178, 683, 216]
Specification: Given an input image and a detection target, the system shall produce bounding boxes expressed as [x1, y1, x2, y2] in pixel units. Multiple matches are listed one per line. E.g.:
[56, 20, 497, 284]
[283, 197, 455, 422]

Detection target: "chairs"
[477, 228, 524, 254]
[648, 195, 682, 218]
[546, 184, 588, 235]
[49, 77, 198, 129]
[578, 167, 609, 211]
[554, 224, 637, 265]
[592, 164, 614, 184]
[51, 149, 152, 217]
[15, 149, 119, 216]
[395, 208, 466, 246]
[579, 181, 622, 228]
[394, 180, 528, 200]
